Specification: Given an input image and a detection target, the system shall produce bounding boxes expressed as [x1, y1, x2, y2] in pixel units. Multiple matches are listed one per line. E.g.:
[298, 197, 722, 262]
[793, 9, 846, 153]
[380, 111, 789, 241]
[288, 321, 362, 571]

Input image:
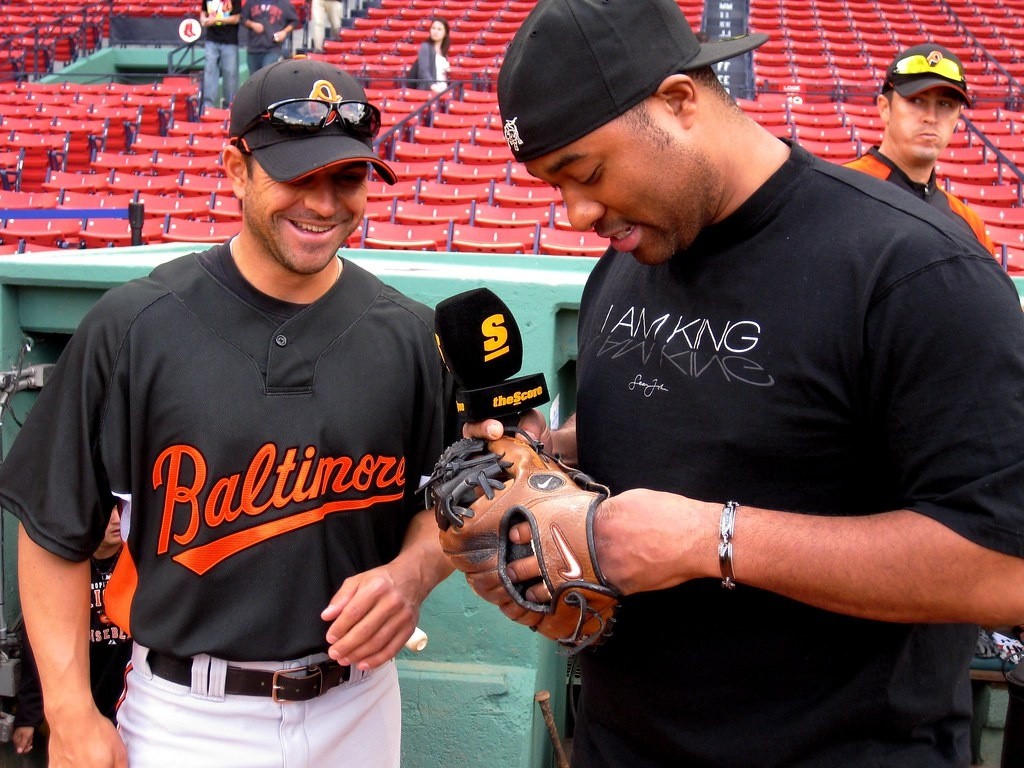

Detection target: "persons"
[1, 60, 462, 768]
[462, 0, 1024, 768]
[417, 18, 449, 93]
[12, 496, 132, 768]
[200, 0, 242, 106]
[241, 0, 342, 75]
[841, 44, 995, 257]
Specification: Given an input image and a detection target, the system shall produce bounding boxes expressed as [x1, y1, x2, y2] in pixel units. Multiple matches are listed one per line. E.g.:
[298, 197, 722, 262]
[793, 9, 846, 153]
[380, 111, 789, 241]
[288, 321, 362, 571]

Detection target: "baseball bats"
[535, 690, 570, 768]
[403, 627, 428, 651]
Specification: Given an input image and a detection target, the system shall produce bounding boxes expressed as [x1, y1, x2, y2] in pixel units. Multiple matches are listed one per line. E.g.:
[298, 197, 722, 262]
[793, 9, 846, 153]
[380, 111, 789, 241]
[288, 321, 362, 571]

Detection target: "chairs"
[0, 1, 1024, 278]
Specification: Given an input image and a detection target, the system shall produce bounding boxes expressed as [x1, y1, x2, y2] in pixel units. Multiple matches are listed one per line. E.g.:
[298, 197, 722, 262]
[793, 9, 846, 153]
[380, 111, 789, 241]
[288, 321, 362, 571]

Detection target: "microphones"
[434, 287, 549, 455]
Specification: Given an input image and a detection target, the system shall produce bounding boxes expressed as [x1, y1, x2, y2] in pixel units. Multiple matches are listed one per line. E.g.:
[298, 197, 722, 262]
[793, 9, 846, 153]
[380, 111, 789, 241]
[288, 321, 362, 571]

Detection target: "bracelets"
[718, 501, 739, 590]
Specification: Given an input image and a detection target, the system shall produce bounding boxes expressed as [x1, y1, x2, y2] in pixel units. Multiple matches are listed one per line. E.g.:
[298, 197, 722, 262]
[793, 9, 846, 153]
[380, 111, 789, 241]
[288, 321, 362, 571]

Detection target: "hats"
[882, 44, 977, 109]
[492, 0, 770, 163]
[226, 55, 400, 188]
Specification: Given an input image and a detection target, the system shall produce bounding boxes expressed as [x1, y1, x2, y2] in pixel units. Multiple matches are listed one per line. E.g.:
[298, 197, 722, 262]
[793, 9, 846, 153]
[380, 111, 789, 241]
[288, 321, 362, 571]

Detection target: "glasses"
[234, 96, 383, 139]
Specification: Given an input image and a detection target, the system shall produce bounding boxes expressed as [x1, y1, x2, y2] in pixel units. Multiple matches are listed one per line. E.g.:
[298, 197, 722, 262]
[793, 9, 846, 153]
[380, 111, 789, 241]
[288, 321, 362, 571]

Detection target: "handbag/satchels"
[406, 58, 419, 89]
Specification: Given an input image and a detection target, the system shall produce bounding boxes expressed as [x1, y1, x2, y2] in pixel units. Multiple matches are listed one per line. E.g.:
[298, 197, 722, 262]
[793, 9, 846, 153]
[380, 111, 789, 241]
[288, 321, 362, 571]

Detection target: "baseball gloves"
[414, 425, 629, 652]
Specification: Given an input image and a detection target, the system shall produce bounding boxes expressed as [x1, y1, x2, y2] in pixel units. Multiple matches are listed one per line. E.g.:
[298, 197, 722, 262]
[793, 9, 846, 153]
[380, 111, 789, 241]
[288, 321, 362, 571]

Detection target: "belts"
[149, 649, 353, 702]
